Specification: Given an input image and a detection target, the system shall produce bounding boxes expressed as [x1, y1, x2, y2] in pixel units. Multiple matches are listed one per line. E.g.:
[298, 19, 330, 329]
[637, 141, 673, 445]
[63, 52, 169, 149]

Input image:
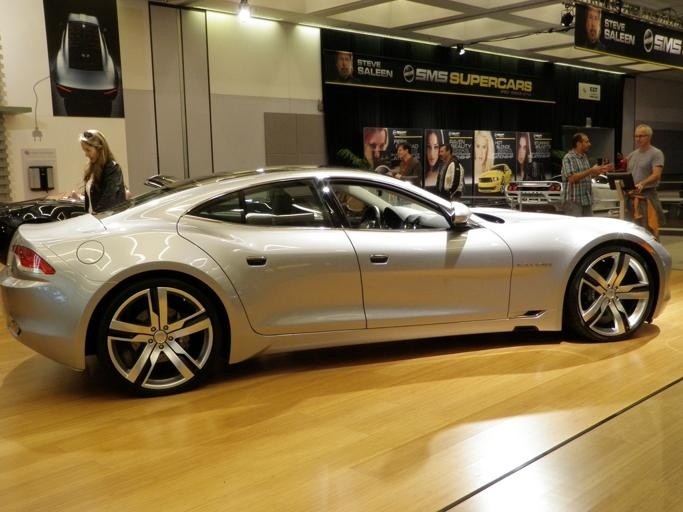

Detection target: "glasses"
[84, 131, 93, 138]
[633, 134, 649, 138]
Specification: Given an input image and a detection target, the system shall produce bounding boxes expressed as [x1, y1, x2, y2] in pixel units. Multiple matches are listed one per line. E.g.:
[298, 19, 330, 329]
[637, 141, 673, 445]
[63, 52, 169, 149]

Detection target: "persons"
[363, 126, 534, 193]
[585, 3, 602, 39]
[68, 129, 128, 215]
[619, 123, 666, 240]
[435, 143, 469, 199]
[331, 50, 352, 80]
[557, 134, 615, 216]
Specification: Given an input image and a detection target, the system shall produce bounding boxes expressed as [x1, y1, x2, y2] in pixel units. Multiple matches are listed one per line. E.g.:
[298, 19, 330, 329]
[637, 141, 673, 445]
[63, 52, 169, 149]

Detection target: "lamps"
[237, 0, 250, 20]
[457, 43, 465, 55]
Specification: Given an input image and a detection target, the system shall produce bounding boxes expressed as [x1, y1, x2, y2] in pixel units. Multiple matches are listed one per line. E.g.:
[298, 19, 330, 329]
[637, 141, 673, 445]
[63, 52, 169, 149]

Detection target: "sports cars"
[55, 12, 119, 102]
[0, 166, 672, 396]
[502, 170, 626, 214]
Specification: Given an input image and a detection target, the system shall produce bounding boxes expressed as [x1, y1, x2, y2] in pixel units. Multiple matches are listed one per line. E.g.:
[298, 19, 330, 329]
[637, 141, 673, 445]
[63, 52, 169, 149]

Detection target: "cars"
[478, 163, 512, 195]
[0, 175, 364, 268]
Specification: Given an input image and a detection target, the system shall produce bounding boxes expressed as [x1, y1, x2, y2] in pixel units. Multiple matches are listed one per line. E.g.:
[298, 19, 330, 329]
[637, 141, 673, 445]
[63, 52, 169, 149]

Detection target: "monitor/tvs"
[608, 172, 635, 191]
